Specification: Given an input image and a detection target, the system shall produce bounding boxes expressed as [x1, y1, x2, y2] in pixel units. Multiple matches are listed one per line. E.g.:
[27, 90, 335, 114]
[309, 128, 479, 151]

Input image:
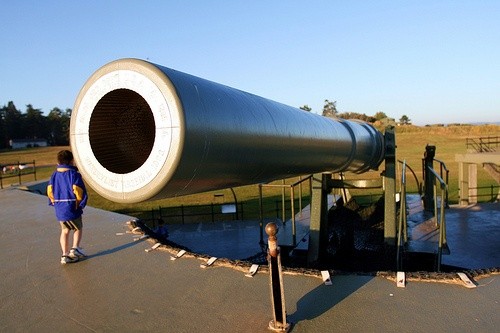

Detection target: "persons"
[46, 149, 88, 265]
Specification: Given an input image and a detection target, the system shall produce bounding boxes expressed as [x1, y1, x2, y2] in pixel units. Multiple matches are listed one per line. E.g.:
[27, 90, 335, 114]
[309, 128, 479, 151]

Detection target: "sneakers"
[61, 255, 79, 263]
[68, 247, 88, 257]
